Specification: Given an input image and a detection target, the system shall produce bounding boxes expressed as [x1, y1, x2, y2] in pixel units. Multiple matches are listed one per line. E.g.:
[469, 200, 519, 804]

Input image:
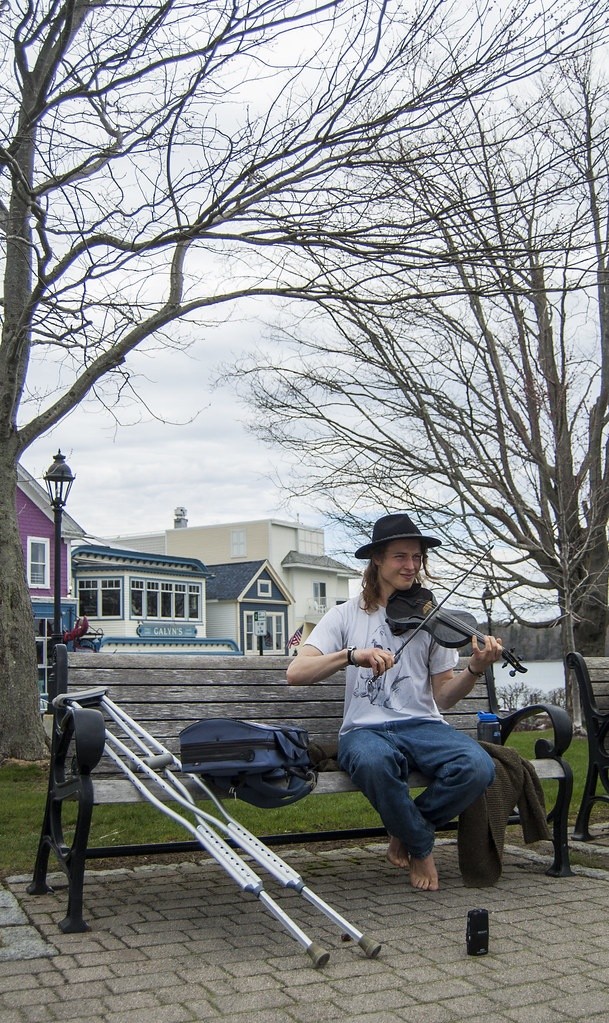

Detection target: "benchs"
[564, 650, 609, 841]
[24, 643, 571, 933]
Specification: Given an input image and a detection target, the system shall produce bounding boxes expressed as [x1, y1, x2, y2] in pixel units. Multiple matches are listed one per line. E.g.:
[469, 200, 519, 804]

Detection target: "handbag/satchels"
[179, 716, 317, 808]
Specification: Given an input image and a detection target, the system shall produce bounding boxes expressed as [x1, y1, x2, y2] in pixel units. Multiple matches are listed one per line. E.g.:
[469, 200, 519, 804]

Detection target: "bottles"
[476, 710, 502, 745]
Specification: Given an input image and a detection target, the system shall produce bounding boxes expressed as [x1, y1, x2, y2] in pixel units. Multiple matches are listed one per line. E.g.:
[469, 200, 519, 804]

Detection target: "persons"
[286, 513, 503, 891]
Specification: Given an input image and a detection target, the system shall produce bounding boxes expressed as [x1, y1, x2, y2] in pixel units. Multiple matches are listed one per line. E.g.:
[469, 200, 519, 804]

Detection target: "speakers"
[466, 907, 489, 956]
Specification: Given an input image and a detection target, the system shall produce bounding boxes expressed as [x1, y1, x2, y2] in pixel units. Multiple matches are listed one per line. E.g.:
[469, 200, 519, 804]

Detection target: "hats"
[354, 514, 441, 559]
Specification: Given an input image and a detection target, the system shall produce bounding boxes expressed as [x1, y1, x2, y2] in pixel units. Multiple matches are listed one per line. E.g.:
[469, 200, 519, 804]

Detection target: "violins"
[385, 581, 528, 678]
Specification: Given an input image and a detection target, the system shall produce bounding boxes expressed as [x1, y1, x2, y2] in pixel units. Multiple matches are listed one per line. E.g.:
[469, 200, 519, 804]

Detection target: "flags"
[286, 623, 304, 649]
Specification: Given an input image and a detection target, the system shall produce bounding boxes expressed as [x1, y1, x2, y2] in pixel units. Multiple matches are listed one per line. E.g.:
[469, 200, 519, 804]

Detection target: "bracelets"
[467, 665, 485, 679]
[347, 645, 356, 665]
[351, 650, 359, 667]
